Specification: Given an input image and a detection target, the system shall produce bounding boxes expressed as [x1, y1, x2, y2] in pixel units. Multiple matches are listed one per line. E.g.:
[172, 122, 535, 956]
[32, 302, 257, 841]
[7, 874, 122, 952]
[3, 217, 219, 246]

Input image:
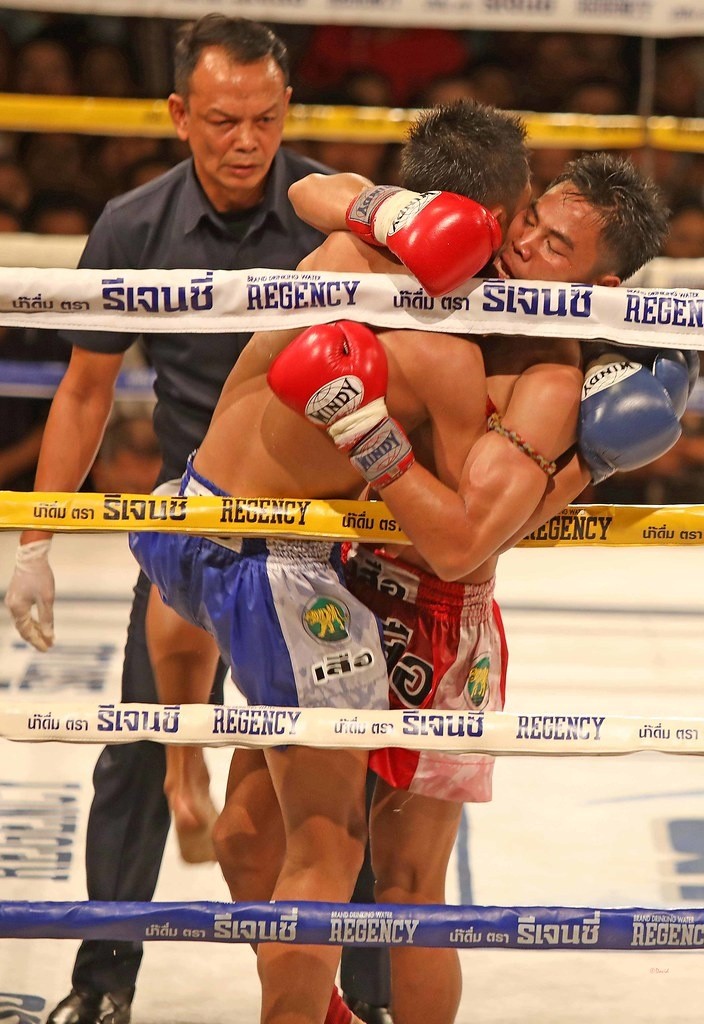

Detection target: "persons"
[0, 43, 704, 523]
[209, 151, 672, 1024]
[127, 94, 700, 1024]
[7, 14, 394, 1024]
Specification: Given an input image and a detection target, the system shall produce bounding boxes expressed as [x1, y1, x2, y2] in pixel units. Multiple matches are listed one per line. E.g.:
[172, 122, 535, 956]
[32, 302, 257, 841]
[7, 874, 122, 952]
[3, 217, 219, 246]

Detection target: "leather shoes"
[343, 991, 392, 1024]
[48, 989, 132, 1024]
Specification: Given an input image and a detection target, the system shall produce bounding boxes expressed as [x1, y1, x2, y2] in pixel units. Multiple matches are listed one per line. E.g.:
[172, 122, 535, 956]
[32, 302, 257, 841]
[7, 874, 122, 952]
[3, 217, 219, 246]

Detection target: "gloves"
[267, 318, 413, 492]
[345, 186, 504, 298]
[581, 362, 684, 487]
[642, 347, 701, 422]
